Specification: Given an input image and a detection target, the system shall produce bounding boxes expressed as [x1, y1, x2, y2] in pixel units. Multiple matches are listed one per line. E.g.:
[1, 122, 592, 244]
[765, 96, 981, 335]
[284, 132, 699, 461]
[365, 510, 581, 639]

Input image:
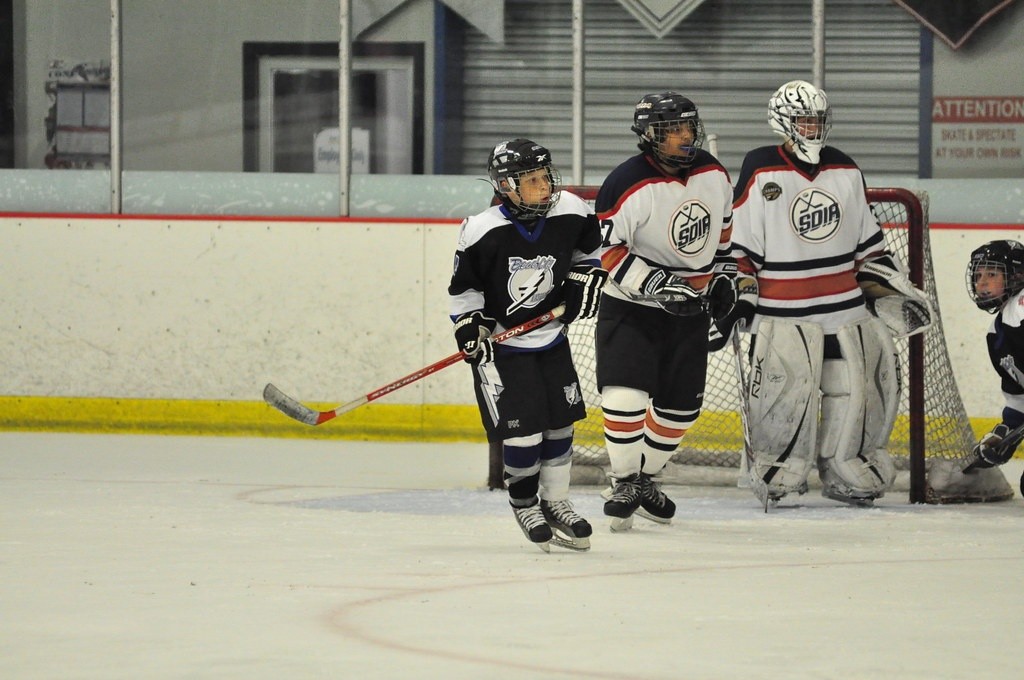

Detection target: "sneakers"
[633, 473, 675, 524]
[512, 503, 554, 553]
[540, 497, 593, 552]
[603, 472, 644, 532]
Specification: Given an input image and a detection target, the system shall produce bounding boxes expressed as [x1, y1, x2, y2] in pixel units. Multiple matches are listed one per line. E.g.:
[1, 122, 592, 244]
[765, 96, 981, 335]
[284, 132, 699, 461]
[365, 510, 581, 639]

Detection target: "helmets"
[488, 138, 562, 220]
[630, 90, 705, 169]
[767, 80, 831, 164]
[964, 240, 1024, 314]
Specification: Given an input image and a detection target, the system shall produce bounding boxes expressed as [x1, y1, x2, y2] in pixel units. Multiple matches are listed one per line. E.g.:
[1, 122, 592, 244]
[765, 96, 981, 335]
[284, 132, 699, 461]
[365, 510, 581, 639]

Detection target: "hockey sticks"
[732, 323, 770, 514]
[961, 422, 1024, 475]
[261, 303, 564, 427]
[608, 275, 712, 303]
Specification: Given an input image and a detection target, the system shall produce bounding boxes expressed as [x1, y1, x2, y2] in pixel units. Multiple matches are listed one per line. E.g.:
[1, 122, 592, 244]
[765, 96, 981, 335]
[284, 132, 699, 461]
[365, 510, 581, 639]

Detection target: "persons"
[708, 80, 935, 515]
[446, 138, 609, 553]
[965, 239, 1024, 496]
[594, 92, 739, 533]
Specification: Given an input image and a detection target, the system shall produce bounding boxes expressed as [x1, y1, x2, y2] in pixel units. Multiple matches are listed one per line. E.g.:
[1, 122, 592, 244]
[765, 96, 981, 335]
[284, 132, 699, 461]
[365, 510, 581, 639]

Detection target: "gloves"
[969, 423, 1023, 470]
[547, 264, 610, 322]
[705, 271, 738, 322]
[639, 267, 704, 317]
[453, 311, 497, 367]
